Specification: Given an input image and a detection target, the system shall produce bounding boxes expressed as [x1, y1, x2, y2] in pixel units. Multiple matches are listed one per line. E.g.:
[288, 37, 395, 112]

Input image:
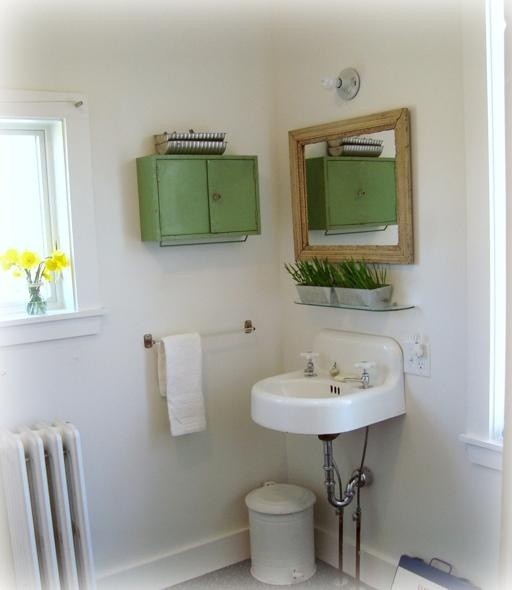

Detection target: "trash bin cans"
[245, 480, 317, 586]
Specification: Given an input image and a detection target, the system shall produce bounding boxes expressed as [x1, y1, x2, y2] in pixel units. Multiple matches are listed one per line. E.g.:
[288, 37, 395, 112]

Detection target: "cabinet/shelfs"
[305, 156, 397, 235]
[136, 154, 261, 243]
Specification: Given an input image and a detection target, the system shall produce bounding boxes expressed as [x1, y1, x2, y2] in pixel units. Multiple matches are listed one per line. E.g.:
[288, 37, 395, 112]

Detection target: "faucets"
[342, 360, 376, 389]
[300, 349, 319, 376]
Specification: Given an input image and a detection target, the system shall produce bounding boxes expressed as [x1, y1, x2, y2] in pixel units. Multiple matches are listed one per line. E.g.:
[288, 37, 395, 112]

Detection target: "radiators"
[0, 419, 97, 590]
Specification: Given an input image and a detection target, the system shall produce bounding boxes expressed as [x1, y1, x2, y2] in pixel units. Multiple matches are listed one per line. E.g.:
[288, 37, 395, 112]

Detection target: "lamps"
[320, 67, 361, 101]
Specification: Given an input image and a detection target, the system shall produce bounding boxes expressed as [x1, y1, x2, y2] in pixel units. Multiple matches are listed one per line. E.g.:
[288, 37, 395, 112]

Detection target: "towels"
[156, 332, 208, 437]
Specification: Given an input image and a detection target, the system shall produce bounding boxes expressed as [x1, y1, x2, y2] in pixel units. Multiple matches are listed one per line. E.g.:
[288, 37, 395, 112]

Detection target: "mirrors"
[286, 107, 415, 265]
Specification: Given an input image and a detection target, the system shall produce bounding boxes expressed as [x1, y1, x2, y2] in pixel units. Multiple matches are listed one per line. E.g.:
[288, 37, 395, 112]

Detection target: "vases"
[334, 284, 391, 306]
[294, 283, 332, 305]
[24, 281, 48, 315]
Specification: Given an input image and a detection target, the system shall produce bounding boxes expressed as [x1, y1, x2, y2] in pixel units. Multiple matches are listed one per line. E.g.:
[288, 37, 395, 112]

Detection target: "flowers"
[0, 247, 69, 314]
[284, 256, 332, 287]
[333, 256, 389, 289]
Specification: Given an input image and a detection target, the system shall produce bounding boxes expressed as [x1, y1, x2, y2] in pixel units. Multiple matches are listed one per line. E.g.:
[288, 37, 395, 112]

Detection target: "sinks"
[250, 329, 408, 436]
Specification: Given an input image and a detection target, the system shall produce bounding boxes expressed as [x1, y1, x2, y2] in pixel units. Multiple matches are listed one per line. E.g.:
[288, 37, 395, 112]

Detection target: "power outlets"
[404, 342, 432, 377]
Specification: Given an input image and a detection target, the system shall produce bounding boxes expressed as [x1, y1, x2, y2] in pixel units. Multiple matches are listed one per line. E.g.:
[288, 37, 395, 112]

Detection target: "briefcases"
[390, 554, 479, 590]
